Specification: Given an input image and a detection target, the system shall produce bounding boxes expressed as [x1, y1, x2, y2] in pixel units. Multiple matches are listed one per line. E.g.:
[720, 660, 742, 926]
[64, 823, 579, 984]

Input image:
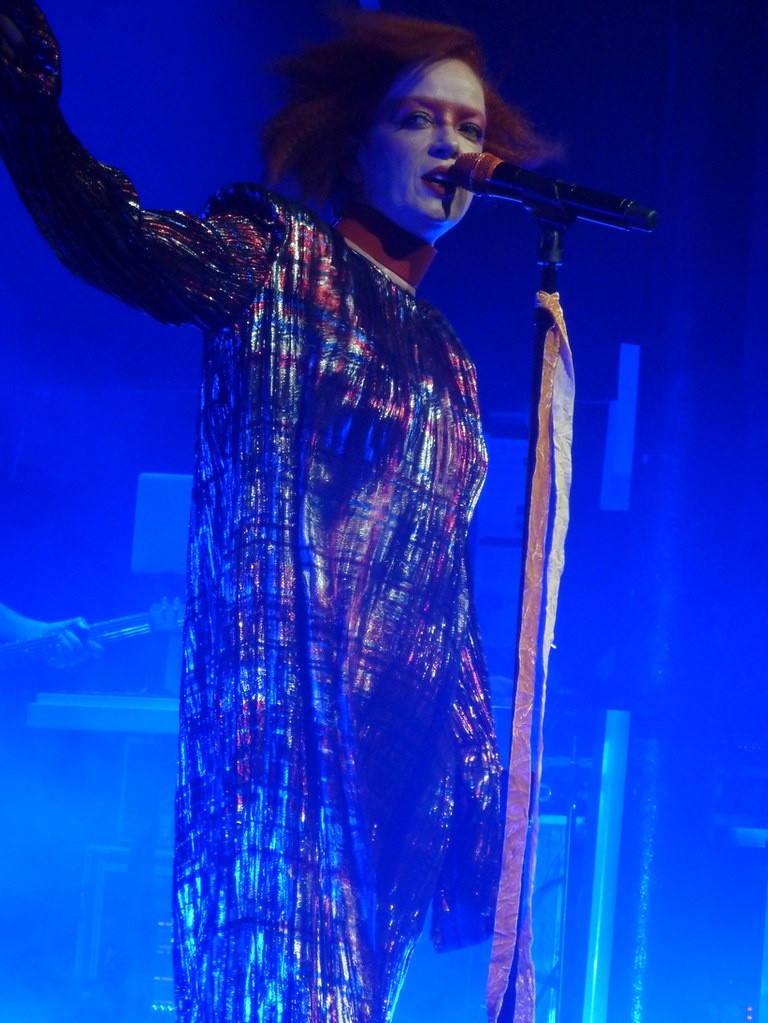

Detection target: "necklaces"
[332, 202, 438, 289]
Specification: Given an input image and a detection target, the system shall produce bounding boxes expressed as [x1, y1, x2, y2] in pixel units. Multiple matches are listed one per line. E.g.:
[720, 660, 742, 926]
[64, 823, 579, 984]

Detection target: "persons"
[0, 0, 544, 1023]
[0, 602, 91, 660]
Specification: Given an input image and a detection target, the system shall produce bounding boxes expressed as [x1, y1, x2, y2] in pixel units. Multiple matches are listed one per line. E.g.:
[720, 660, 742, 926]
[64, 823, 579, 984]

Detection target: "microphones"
[454, 152, 659, 233]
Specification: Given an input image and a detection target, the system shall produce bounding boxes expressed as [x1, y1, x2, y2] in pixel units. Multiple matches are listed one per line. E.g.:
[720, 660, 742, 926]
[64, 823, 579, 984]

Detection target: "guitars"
[0, 597, 184, 675]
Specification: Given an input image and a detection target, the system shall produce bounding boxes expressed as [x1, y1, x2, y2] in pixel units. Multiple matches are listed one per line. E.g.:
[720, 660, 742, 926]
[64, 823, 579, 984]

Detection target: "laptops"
[131, 473, 194, 575]
